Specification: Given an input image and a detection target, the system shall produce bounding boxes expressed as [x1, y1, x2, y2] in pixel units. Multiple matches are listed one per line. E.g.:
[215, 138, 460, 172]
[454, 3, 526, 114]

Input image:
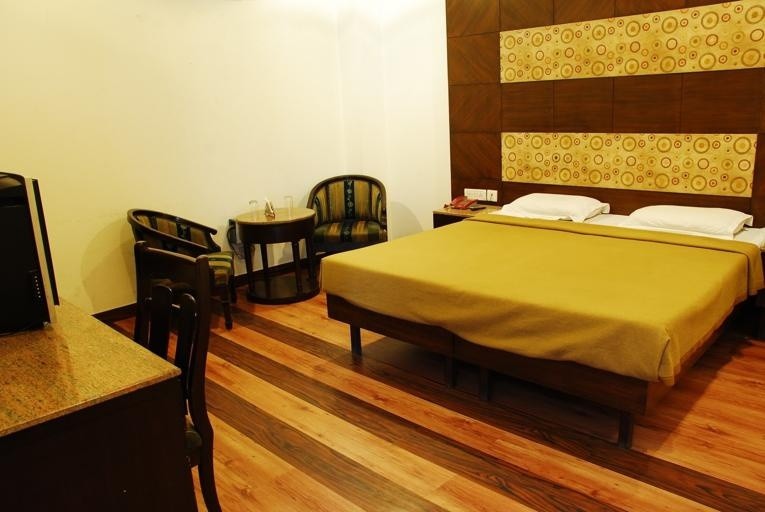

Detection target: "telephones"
[448, 195, 479, 210]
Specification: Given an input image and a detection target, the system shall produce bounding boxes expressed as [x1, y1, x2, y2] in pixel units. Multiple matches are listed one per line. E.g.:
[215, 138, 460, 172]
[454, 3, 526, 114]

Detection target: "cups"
[249, 200, 259, 220]
[284, 196, 293, 220]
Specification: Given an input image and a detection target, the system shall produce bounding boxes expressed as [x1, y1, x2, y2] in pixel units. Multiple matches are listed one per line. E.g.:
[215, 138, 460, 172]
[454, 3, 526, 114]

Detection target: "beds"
[315, 210, 765, 449]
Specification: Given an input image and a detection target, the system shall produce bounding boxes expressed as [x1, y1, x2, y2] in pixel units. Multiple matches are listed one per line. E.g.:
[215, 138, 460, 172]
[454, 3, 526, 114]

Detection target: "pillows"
[487, 192, 611, 223]
[621, 204, 754, 240]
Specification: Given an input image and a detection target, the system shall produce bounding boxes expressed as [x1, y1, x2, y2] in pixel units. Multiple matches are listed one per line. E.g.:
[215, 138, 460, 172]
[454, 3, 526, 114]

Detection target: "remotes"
[468, 205, 486, 211]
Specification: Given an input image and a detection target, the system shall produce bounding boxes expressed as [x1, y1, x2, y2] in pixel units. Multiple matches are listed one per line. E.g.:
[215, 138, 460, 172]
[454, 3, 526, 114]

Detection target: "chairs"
[306, 175, 387, 260]
[133, 239, 222, 512]
[127, 209, 236, 330]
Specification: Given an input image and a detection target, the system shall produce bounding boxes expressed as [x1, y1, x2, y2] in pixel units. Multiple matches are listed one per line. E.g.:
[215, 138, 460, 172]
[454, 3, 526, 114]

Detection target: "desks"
[228, 206, 320, 305]
[0, 296, 199, 512]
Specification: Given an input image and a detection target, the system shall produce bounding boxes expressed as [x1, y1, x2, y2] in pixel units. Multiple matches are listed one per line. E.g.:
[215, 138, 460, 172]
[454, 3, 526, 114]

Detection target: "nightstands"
[433, 205, 501, 227]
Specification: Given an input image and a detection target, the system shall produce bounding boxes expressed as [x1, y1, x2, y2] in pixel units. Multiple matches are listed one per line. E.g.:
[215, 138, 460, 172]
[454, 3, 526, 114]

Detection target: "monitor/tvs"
[0, 170, 63, 338]
